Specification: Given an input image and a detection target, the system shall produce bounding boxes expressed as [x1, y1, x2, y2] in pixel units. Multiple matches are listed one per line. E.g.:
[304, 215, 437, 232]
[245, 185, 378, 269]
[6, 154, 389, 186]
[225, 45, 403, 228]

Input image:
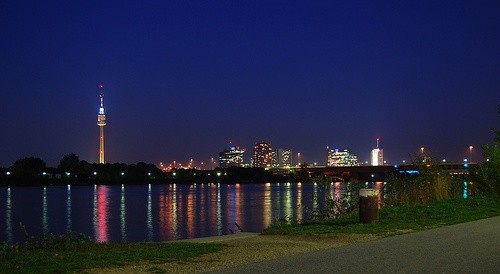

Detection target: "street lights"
[469, 145, 473, 164]
